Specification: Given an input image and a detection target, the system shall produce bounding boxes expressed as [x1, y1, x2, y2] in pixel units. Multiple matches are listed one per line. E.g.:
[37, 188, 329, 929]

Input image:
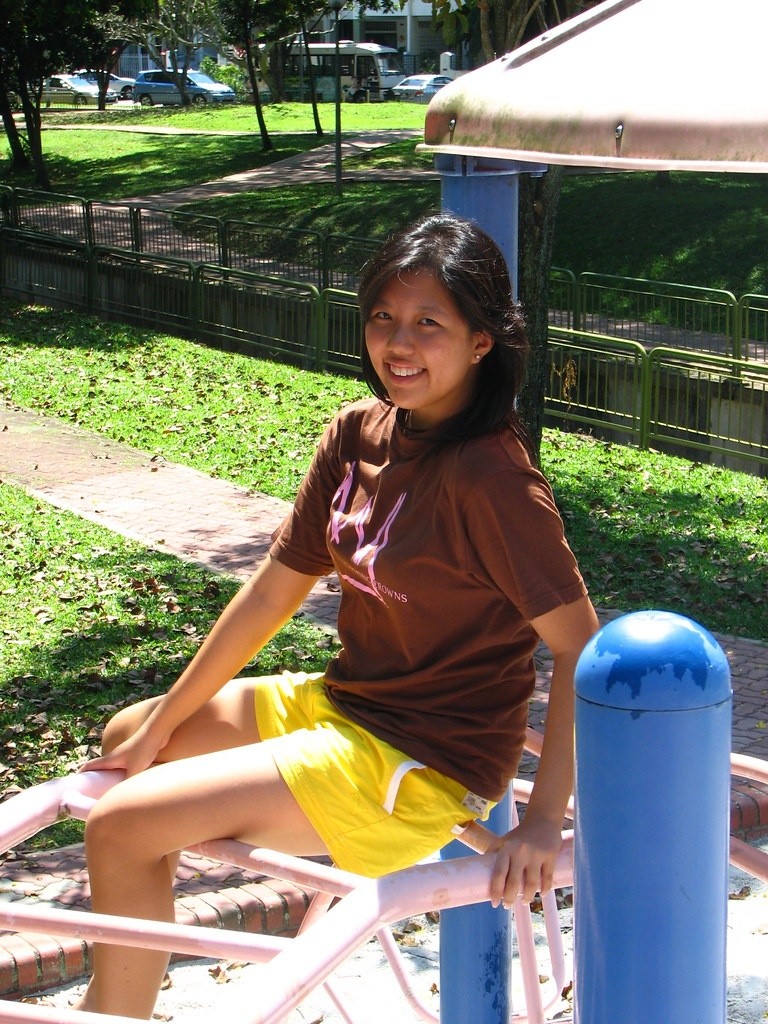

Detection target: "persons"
[76, 215, 601, 1020]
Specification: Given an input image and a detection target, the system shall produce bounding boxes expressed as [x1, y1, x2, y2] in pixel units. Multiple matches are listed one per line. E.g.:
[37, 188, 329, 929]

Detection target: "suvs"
[132, 69, 235, 107]
[73, 69, 135, 100]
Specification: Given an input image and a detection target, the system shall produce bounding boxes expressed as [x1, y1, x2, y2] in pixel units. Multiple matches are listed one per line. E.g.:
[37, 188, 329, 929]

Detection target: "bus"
[246, 39, 407, 103]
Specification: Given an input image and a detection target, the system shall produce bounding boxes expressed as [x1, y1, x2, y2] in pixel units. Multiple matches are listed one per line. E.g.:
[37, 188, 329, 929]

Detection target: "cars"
[41, 74, 119, 106]
[392, 74, 454, 104]
